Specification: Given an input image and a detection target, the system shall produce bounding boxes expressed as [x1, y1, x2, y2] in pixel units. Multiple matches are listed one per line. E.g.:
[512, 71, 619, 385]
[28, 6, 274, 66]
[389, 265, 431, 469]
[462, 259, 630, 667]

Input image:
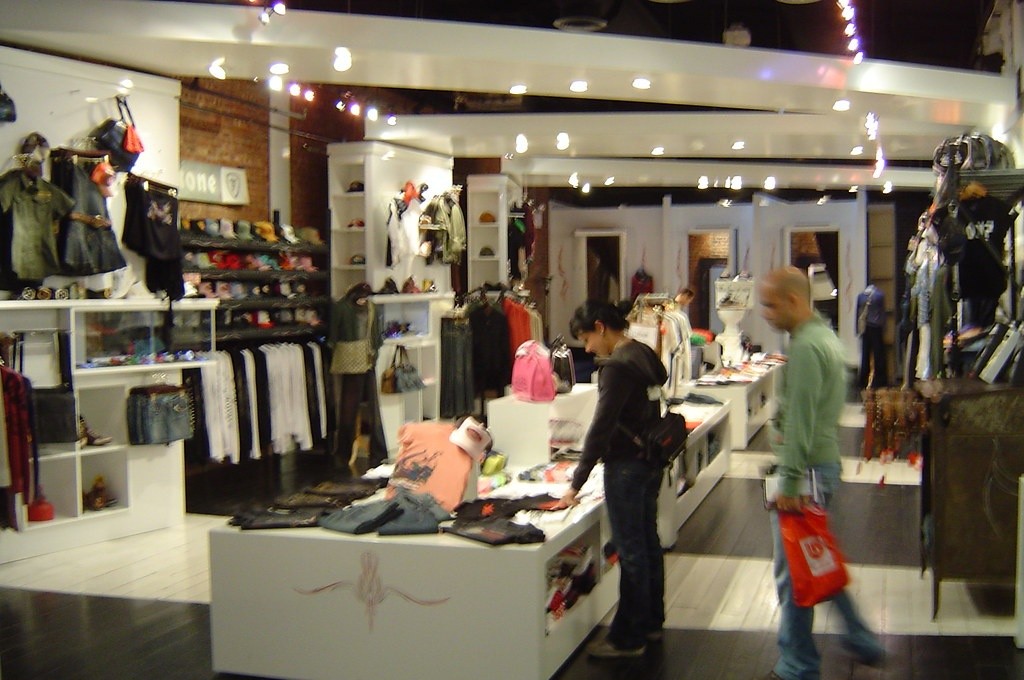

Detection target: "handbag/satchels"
[858, 298, 872, 337]
[30, 382, 78, 444]
[381, 345, 427, 393]
[28, 494, 54, 521]
[0, 85, 16, 124]
[89, 119, 145, 174]
[812, 281, 836, 301]
[549, 333, 576, 394]
[863, 389, 922, 465]
[932, 130, 1016, 174]
[778, 494, 850, 608]
[648, 411, 688, 465]
[330, 339, 378, 375]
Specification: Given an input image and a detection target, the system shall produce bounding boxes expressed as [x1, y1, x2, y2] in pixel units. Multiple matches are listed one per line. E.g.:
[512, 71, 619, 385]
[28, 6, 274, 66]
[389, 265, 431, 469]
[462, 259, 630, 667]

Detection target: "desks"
[656, 401, 733, 553]
[904, 374, 1024, 621]
[686, 363, 783, 449]
[208, 467, 618, 680]
[486, 384, 598, 469]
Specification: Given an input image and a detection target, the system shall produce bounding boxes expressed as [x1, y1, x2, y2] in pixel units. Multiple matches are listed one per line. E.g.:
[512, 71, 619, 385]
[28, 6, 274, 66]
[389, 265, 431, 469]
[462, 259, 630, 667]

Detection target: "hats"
[346, 181, 364, 192]
[480, 247, 495, 256]
[348, 218, 364, 228]
[350, 255, 365, 265]
[479, 213, 495, 223]
[179, 217, 324, 330]
[91, 163, 115, 197]
[22, 133, 50, 178]
[449, 416, 492, 463]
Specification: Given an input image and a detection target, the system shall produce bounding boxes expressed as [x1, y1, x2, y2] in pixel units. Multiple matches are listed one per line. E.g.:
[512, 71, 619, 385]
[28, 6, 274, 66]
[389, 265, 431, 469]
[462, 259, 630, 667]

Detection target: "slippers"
[82, 477, 118, 511]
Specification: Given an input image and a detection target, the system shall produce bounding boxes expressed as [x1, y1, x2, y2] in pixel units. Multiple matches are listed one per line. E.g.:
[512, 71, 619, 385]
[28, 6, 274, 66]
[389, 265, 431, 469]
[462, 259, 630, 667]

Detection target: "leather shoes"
[764, 669, 821, 680]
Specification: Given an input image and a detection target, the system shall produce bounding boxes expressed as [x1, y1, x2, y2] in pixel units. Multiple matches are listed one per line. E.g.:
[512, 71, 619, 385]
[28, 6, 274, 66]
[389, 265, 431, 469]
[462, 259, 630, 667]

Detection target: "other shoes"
[642, 631, 664, 641]
[585, 639, 645, 657]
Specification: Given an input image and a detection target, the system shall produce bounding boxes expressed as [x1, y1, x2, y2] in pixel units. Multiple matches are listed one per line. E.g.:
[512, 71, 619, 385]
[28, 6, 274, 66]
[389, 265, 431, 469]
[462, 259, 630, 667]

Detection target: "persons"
[549, 295, 668, 658]
[668, 286, 695, 313]
[754, 266, 890, 680]
[854, 284, 888, 394]
[630, 266, 653, 300]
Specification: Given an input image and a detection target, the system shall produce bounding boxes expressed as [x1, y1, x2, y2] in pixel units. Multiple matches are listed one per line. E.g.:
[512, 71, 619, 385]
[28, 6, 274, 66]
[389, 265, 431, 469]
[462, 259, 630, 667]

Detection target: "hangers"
[195, 328, 315, 358]
[0, 358, 27, 383]
[13, 153, 37, 179]
[131, 371, 184, 396]
[69, 155, 111, 166]
[435, 186, 463, 203]
[628, 291, 682, 327]
[452, 288, 542, 320]
[128, 174, 179, 198]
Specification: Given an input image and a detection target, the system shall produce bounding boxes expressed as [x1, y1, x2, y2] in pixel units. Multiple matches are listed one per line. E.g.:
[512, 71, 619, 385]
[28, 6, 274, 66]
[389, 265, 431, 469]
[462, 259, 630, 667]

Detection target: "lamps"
[259, 0, 287, 25]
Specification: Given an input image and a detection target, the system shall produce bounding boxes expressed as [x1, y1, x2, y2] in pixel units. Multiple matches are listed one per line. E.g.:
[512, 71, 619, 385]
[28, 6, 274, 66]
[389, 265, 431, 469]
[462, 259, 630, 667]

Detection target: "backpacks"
[511, 340, 558, 402]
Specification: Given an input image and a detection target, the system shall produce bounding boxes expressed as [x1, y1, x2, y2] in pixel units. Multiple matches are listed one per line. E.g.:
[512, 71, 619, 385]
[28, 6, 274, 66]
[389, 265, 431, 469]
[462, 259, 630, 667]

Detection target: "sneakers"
[78, 413, 113, 449]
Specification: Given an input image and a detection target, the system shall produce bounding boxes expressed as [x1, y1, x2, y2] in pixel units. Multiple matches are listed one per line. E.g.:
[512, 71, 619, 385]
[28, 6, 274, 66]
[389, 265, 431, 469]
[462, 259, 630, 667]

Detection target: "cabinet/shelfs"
[328, 144, 455, 454]
[178, 237, 327, 342]
[0, 290, 217, 567]
[467, 172, 528, 290]
